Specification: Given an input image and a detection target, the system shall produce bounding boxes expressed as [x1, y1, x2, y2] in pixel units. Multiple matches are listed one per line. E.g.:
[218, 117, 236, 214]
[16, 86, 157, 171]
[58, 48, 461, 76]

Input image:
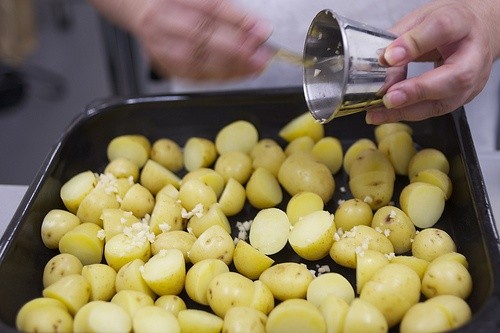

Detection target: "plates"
[0, 85, 500, 333]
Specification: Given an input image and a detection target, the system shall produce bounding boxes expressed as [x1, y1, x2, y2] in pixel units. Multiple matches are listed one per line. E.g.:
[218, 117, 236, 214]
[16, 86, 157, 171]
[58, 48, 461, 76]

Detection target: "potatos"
[16, 108, 472, 333]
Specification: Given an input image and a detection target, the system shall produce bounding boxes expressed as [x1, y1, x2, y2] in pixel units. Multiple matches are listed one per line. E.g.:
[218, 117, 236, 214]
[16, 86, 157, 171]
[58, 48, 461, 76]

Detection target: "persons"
[89, 0, 500, 126]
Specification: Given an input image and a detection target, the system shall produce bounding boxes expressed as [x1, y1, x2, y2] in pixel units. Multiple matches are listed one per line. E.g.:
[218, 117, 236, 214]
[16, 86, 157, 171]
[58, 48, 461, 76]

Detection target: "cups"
[302, 9, 407, 124]
